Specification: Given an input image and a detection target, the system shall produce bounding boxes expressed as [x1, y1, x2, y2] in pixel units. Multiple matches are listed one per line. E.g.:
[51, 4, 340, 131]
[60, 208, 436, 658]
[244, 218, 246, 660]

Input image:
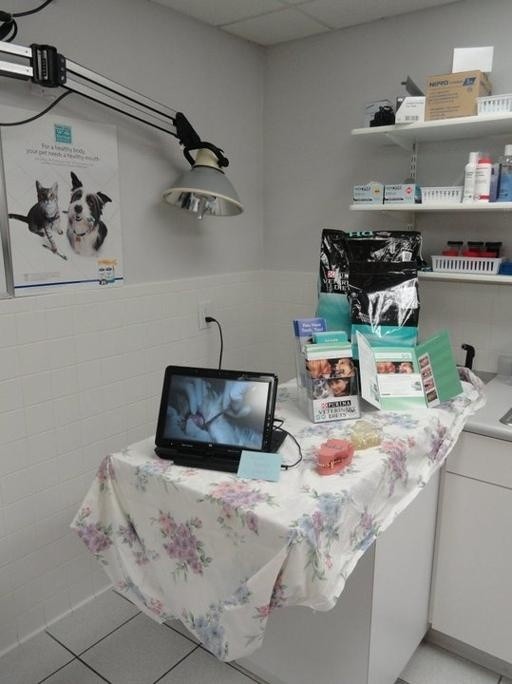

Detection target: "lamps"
[0, 40, 244, 218]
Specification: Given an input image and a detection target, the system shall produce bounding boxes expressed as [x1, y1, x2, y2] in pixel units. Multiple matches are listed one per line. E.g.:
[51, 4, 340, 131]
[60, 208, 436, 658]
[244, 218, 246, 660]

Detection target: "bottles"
[441, 239, 503, 257]
[461, 143, 512, 203]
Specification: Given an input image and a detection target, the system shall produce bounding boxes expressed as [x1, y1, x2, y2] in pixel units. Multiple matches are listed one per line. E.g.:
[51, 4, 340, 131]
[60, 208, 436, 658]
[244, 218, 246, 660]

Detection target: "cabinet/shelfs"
[70, 364, 511, 684]
[349, 111, 512, 283]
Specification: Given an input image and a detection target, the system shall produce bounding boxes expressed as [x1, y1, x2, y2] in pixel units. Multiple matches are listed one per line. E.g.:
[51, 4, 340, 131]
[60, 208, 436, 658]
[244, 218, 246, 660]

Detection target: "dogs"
[67, 170, 113, 257]
[335, 358, 355, 377]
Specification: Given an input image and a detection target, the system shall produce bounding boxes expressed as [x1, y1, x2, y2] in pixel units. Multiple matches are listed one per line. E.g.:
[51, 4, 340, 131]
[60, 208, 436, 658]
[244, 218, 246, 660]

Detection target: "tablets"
[155, 365, 278, 456]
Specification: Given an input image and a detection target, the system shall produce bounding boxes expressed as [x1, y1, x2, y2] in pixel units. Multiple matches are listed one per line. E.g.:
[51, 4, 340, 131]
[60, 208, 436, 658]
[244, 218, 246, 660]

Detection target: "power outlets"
[197, 299, 215, 330]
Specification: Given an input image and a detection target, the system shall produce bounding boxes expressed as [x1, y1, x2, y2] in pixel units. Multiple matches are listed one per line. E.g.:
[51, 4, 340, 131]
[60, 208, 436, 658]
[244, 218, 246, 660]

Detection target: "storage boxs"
[394, 95, 426, 123]
[425, 69, 492, 122]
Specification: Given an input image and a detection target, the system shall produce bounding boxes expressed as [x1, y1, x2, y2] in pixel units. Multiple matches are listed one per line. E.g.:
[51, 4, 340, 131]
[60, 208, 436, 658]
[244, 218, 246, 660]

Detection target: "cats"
[7, 180, 64, 254]
